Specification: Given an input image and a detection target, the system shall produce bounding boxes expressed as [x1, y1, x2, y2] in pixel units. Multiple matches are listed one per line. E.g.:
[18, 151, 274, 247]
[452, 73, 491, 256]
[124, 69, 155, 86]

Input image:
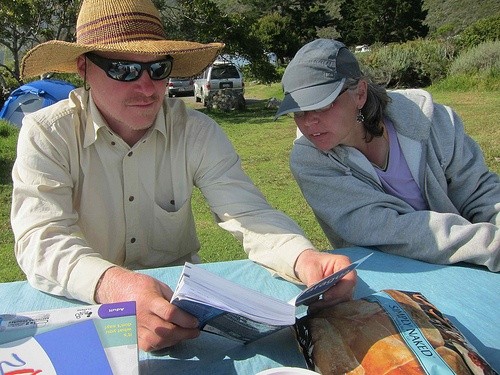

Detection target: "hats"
[19, 0, 226, 81]
[273, 38, 362, 122]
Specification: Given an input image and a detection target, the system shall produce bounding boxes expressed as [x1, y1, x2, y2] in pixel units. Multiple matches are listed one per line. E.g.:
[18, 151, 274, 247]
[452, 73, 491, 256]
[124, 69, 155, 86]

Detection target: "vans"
[193, 62, 245, 106]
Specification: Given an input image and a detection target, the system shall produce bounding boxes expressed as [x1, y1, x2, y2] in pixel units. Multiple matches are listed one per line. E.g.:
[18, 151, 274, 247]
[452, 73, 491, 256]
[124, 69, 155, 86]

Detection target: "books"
[168, 253, 375, 346]
[0, 300, 139, 375]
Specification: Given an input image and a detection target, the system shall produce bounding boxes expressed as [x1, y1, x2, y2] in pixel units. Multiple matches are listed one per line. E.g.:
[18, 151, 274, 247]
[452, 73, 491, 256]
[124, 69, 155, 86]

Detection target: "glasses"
[84, 50, 174, 82]
[283, 80, 354, 118]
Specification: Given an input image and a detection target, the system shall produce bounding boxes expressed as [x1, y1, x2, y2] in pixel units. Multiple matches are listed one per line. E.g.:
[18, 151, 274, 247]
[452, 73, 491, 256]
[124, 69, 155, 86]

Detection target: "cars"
[168, 76, 194, 96]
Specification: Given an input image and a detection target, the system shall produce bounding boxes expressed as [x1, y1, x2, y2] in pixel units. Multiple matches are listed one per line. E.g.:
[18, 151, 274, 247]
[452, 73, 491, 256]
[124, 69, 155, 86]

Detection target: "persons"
[9, 0, 357, 352]
[273, 38, 500, 273]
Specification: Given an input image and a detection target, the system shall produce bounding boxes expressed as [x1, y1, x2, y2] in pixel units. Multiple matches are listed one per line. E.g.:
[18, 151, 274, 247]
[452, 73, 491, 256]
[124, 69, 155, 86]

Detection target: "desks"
[0, 247, 500, 375]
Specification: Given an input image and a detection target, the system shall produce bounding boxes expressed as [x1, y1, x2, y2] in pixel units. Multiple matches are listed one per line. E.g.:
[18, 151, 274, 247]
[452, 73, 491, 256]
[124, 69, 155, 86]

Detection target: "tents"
[0, 80, 76, 125]
